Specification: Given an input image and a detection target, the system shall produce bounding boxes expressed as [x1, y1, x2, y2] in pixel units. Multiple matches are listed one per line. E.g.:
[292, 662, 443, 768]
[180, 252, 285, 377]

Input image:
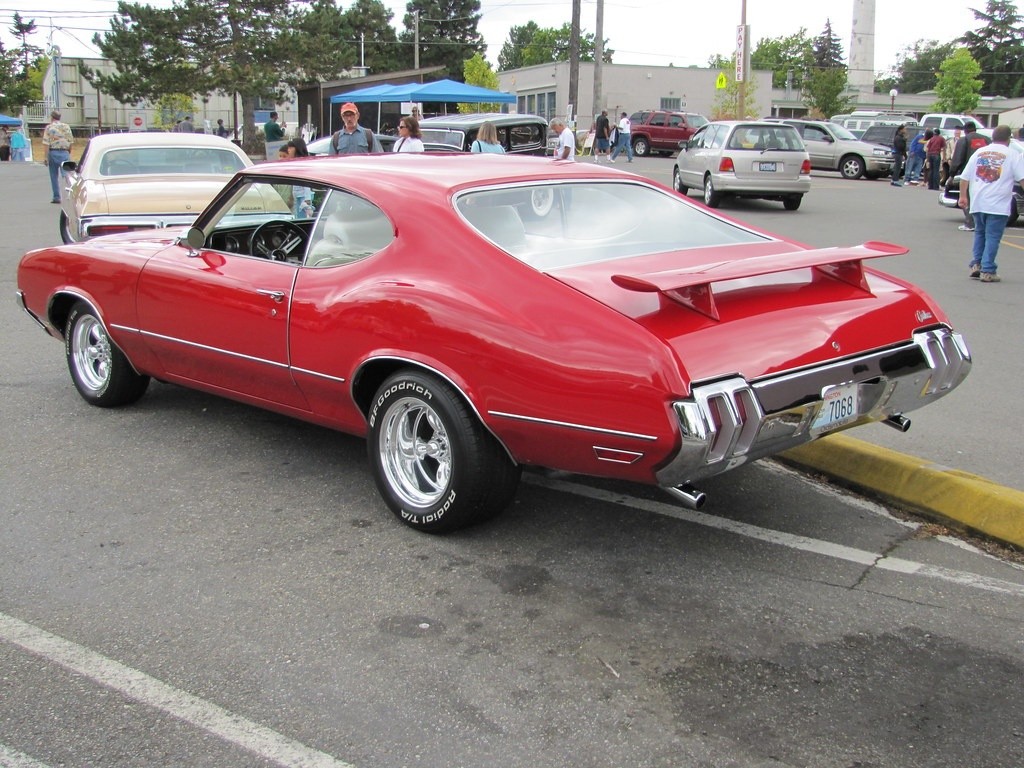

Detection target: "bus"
[829, 111, 919, 139]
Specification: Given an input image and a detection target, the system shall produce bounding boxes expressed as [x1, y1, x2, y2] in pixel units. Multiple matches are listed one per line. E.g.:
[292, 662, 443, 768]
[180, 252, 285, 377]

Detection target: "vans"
[225, 121, 299, 147]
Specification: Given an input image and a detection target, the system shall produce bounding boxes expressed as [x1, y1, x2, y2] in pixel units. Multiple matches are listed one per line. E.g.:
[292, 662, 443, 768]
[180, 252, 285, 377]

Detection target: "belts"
[49, 148, 66, 151]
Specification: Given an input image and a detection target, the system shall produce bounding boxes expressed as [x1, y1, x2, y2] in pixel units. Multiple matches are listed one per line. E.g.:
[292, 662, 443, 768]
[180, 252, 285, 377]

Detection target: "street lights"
[889, 89, 898, 112]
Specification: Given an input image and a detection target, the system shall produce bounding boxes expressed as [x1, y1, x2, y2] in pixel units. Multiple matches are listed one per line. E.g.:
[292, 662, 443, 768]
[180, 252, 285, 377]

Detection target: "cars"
[298, 134, 400, 156]
[394, 113, 549, 158]
[547, 130, 590, 156]
[672, 120, 812, 210]
[938, 139, 1024, 226]
[16, 153, 973, 533]
[59, 132, 296, 244]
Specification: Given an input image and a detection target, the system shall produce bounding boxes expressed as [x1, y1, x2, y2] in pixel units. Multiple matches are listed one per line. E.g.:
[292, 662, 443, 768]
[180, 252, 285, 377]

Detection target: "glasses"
[413, 110, 418, 112]
[341, 113, 357, 118]
[399, 125, 406, 130]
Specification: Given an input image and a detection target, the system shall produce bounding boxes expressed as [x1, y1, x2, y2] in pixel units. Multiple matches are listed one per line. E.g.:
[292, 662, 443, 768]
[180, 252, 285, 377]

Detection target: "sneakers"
[970, 264, 981, 277]
[980, 272, 1002, 280]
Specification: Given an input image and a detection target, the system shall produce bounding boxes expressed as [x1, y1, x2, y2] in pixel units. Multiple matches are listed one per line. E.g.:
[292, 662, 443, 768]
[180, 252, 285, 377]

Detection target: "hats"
[340, 102, 359, 116]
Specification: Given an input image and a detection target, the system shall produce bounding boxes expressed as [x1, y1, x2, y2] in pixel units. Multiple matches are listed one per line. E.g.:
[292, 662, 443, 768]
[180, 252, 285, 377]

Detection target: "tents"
[0, 114, 24, 126]
[330, 79, 516, 135]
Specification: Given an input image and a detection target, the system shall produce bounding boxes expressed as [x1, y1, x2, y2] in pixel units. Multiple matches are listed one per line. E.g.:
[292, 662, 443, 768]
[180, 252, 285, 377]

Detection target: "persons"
[945, 122, 992, 231]
[958, 125, 1024, 283]
[409, 106, 423, 120]
[610, 112, 633, 163]
[904, 121, 961, 189]
[471, 121, 505, 153]
[392, 116, 424, 152]
[287, 138, 315, 220]
[550, 118, 575, 224]
[263, 111, 287, 142]
[215, 119, 226, 138]
[42, 110, 73, 203]
[272, 144, 293, 208]
[329, 103, 384, 155]
[171, 116, 195, 133]
[593, 110, 611, 164]
[0, 125, 10, 161]
[890, 125, 907, 186]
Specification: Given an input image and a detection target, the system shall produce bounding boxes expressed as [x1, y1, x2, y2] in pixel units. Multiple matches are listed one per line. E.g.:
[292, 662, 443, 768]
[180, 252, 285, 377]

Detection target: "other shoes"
[958, 225, 976, 230]
[890, 179, 941, 190]
[594, 158, 633, 164]
[51, 200, 62, 204]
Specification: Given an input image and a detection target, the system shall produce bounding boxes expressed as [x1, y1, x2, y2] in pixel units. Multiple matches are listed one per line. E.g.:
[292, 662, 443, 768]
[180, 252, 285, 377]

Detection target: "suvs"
[919, 114, 1014, 144]
[743, 118, 905, 180]
[860, 124, 949, 177]
[629, 110, 711, 157]
